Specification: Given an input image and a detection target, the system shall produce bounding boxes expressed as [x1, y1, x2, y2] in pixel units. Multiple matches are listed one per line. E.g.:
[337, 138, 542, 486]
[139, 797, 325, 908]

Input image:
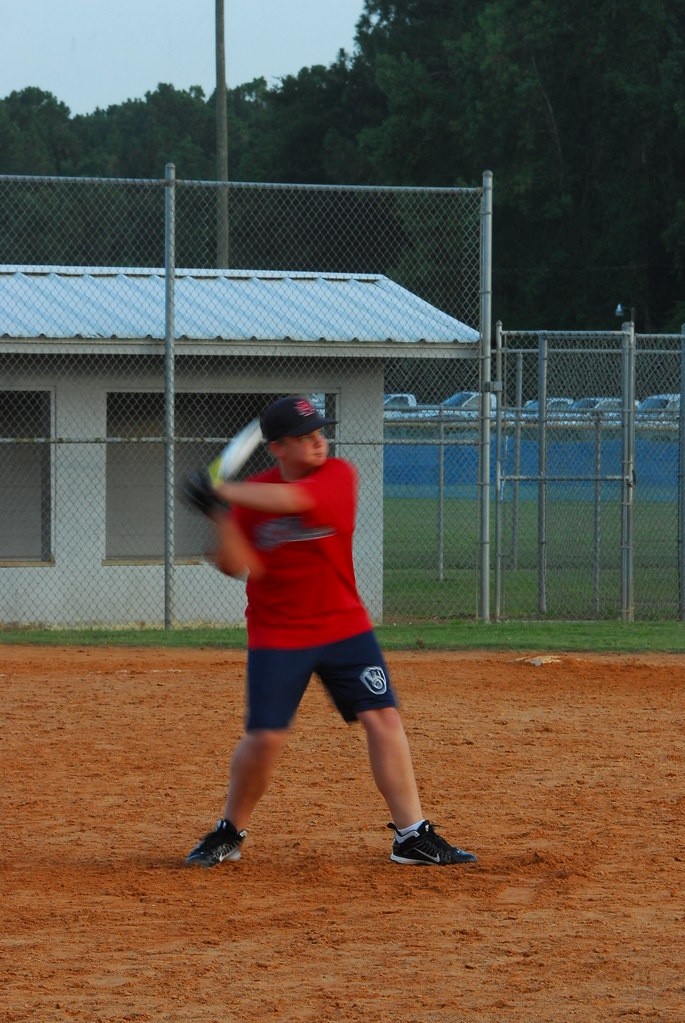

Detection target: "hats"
[260, 397, 339, 442]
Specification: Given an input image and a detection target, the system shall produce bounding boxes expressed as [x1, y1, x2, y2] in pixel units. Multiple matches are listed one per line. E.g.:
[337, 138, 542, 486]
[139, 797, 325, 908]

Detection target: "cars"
[383, 391, 683, 430]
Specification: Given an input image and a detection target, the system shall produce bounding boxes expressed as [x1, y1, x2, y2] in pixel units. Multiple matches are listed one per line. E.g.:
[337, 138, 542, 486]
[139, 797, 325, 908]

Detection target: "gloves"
[180, 467, 224, 515]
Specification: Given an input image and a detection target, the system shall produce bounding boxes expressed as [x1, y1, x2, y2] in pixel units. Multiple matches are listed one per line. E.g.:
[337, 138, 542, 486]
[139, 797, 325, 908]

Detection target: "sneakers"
[187, 819, 248, 866]
[386, 820, 477, 864]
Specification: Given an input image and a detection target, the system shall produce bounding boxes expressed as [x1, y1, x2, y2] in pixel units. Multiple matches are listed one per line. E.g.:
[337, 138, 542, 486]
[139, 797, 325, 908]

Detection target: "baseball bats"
[206, 421, 265, 476]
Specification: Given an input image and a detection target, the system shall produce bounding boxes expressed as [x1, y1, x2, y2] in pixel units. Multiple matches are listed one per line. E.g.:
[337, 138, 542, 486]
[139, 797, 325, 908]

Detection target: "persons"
[184, 394, 480, 870]
